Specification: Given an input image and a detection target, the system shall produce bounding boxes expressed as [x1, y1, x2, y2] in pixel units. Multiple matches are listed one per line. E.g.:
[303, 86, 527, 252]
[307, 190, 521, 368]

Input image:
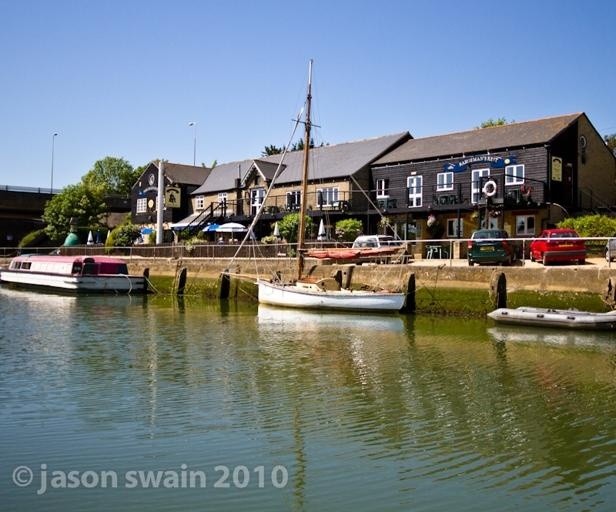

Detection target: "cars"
[351, 234, 409, 264]
[530, 228, 588, 264]
[467, 229, 518, 266]
[604, 232, 616, 263]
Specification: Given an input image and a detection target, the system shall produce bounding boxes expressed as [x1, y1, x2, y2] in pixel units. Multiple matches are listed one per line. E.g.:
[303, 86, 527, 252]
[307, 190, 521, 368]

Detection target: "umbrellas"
[318, 219, 326, 248]
[273, 221, 280, 236]
[216, 222, 248, 245]
[202, 223, 220, 242]
[87, 230, 94, 247]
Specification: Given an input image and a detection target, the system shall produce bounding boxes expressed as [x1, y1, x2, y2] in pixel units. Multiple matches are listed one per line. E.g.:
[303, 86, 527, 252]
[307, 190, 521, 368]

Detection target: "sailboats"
[214, 57, 410, 319]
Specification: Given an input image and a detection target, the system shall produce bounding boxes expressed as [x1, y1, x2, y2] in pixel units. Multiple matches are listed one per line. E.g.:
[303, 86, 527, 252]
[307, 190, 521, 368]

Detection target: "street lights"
[188, 122, 197, 166]
[49, 131, 60, 198]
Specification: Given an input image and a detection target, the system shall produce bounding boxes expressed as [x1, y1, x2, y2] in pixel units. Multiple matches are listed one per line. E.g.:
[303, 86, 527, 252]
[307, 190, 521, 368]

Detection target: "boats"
[486, 302, 616, 331]
[0, 252, 146, 296]
